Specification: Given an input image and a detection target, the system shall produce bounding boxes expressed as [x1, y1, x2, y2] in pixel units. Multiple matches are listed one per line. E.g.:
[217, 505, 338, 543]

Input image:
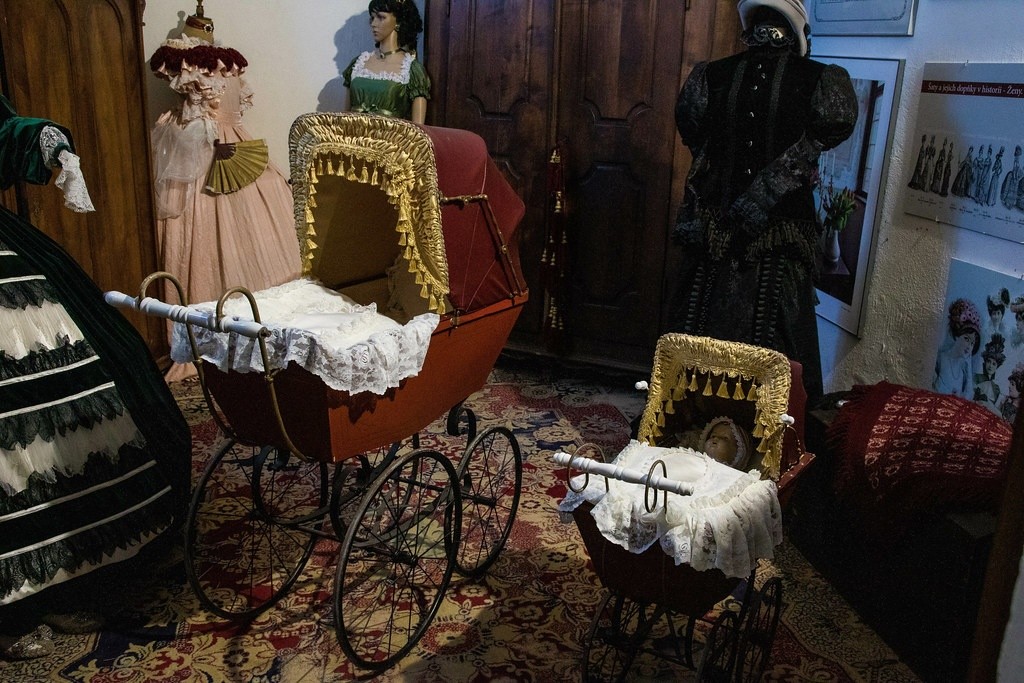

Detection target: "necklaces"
[186, 15, 214, 35]
[380, 48, 403, 59]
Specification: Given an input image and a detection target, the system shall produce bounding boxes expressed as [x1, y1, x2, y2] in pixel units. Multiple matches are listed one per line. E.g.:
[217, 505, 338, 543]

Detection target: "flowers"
[822, 186, 859, 233]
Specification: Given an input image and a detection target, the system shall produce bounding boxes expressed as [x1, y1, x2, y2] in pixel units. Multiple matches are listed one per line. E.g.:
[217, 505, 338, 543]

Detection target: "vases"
[827, 229, 840, 263]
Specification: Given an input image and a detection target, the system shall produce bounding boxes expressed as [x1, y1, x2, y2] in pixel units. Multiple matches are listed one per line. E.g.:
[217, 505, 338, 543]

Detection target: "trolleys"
[534, 333, 816, 683]
[99, 110, 524, 671]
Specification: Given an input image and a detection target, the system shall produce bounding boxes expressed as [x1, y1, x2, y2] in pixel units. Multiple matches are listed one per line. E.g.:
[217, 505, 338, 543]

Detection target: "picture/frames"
[808, 55, 907, 339]
[810, 0, 919, 36]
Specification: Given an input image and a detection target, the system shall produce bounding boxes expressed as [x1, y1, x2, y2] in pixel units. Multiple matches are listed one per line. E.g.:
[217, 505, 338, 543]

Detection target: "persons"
[698, 416, 753, 469]
[342, 0, 431, 124]
[674, 0, 859, 509]
[0, 94, 192, 657]
[150, 1, 302, 383]
